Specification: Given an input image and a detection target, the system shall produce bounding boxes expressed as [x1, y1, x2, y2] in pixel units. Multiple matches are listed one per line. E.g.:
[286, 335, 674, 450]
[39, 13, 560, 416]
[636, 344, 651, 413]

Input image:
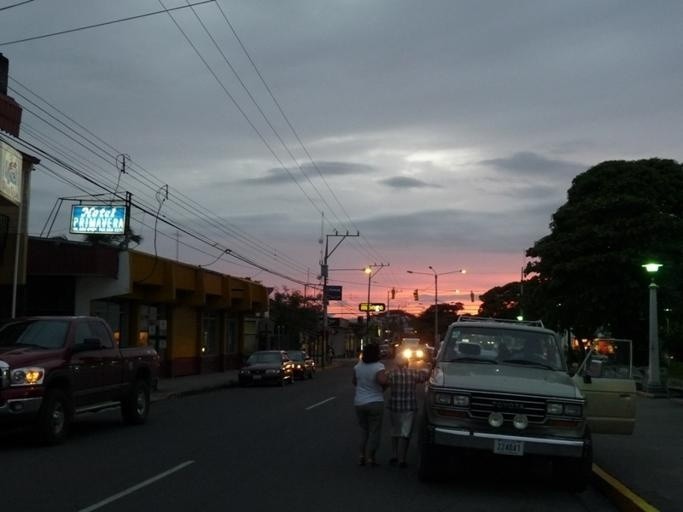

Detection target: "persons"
[385, 350, 434, 465]
[350, 342, 390, 466]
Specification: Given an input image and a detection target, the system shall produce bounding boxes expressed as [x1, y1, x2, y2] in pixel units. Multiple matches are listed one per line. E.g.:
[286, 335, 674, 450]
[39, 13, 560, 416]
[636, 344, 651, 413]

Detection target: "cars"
[285, 348, 319, 380]
[370, 329, 496, 370]
[238, 348, 297, 387]
[587, 350, 608, 365]
[569, 358, 642, 390]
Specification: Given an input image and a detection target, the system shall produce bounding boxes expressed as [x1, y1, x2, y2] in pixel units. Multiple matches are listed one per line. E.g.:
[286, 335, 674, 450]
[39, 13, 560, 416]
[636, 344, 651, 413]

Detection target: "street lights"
[407, 265, 468, 355]
[317, 267, 372, 365]
[639, 254, 670, 399]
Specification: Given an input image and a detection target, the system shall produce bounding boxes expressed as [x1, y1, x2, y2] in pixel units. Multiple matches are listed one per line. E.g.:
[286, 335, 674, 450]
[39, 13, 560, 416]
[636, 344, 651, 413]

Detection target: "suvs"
[418, 312, 637, 493]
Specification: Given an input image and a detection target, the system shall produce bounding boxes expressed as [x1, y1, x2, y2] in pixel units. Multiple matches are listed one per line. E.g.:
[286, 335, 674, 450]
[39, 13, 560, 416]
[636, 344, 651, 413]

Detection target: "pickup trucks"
[0, 311, 161, 446]
